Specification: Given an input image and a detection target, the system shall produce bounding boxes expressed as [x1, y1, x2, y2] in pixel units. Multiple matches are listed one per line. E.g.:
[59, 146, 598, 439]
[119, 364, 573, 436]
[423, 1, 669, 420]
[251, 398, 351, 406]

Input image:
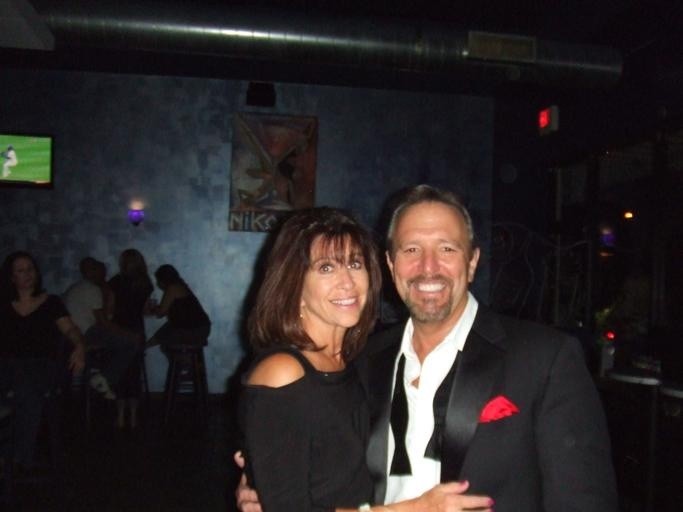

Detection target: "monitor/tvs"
[0, 131, 57, 189]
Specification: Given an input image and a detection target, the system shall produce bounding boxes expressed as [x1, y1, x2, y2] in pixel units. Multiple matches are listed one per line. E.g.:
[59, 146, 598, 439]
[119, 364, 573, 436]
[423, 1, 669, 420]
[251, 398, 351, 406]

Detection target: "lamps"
[128, 199, 145, 226]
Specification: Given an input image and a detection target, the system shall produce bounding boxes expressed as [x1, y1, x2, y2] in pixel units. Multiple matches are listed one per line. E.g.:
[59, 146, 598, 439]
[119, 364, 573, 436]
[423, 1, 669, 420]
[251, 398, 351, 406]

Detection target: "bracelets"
[357, 502, 372, 510]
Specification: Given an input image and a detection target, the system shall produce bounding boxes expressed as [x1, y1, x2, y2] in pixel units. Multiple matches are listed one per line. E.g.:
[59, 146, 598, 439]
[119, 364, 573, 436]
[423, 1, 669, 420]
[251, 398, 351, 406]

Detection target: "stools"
[0, 340, 208, 512]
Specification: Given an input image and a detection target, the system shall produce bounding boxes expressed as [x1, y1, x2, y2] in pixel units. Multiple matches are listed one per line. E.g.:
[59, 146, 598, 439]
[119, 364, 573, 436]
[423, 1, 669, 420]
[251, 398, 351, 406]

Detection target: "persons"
[2, 249, 210, 474]
[235, 183, 618, 511]
[239, 207, 494, 511]
[236, 125, 312, 212]
